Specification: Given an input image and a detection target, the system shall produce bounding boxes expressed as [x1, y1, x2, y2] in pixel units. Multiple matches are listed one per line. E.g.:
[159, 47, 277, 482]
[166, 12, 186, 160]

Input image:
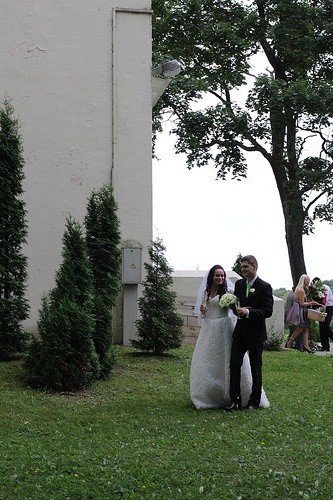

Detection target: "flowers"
[218, 292, 238, 318]
[310, 277, 328, 303]
[249, 288, 256, 293]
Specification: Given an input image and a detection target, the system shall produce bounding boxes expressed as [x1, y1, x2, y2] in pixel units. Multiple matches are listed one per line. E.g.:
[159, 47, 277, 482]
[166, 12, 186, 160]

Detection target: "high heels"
[303, 346, 315, 353]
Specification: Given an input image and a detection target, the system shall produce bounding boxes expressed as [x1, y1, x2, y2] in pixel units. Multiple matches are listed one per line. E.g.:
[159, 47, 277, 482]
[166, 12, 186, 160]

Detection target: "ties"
[245, 284, 251, 298]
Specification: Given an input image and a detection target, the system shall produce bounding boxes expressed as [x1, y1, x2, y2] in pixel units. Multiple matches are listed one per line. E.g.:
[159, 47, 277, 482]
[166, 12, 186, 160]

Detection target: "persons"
[285, 274, 333, 353]
[190, 265, 269, 409]
[223, 256, 274, 412]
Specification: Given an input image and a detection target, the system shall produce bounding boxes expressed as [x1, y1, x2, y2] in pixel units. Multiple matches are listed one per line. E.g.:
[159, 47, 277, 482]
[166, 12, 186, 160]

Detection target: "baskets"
[307, 303, 328, 322]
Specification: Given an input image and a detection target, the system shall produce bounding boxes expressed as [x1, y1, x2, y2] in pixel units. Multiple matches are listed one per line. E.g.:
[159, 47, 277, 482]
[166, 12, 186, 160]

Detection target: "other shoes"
[319, 347, 330, 351]
[223, 401, 243, 412]
[241, 403, 260, 411]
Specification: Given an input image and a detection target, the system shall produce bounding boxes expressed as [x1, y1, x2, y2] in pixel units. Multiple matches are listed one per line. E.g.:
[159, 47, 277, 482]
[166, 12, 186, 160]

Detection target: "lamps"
[152, 58, 183, 79]
